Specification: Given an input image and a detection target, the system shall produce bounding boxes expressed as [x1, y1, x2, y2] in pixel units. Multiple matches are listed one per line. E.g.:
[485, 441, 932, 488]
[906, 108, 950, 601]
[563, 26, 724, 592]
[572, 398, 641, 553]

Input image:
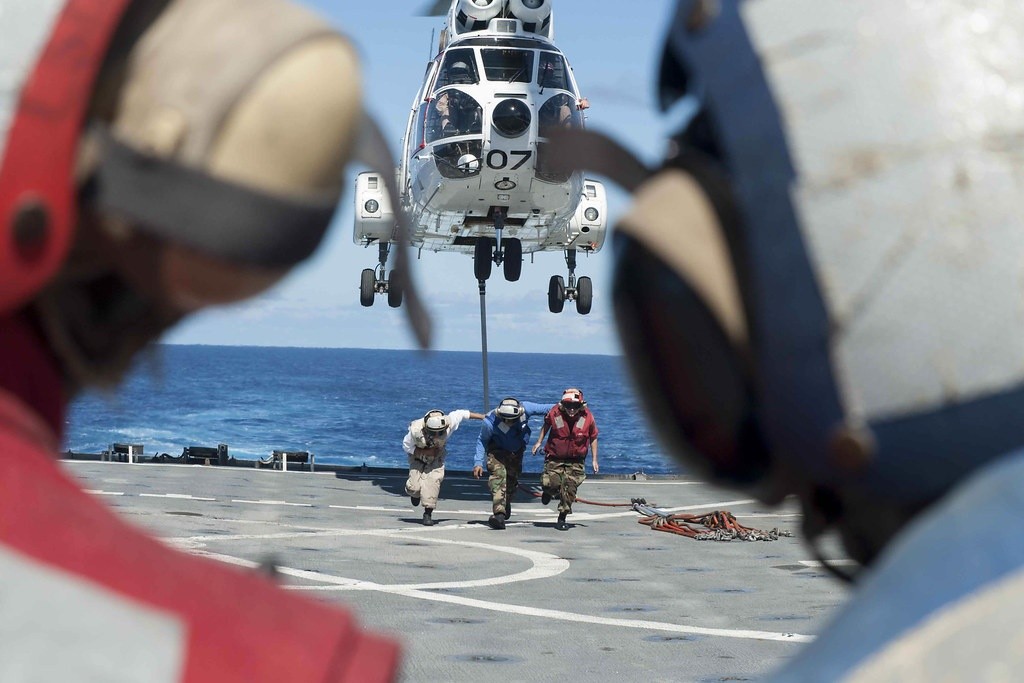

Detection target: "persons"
[474, 397, 556, 530]
[535, 0, 1024, 683]
[0, 0, 439, 683]
[530, 60, 563, 138]
[402, 409, 485, 526]
[436, 62, 481, 155]
[532, 388, 599, 531]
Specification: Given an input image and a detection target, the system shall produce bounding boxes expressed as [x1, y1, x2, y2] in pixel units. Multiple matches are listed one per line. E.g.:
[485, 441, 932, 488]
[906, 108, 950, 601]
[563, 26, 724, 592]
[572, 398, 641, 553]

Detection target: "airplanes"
[352, 0, 608, 317]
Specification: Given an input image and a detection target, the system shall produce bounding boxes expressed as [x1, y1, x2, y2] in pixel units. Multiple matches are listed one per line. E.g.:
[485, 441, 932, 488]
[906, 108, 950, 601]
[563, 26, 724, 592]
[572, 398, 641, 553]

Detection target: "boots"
[489, 513, 505, 529]
[542, 492, 551, 505]
[423, 507, 434, 526]
[505, 497, 511, 519]
[555, 512, 569, 530]
[411, 496, 420, 506]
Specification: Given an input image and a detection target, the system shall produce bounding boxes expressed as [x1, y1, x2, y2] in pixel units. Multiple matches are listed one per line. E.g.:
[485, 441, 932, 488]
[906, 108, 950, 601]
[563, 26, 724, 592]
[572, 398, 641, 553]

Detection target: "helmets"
[424, 410, 448, 431]
[593, 3, 1023, 530]
[0, 0, 366, 387]
[497, 397, 521, 419]
[560, 388, 584, 404]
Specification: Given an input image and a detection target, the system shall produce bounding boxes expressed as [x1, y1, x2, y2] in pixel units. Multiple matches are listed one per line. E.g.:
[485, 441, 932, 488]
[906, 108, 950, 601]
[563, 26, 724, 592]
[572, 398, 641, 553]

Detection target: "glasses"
[502, 418, 519, 423]
[427, 429, 445, 437]
[563, 402, 582, 409]
